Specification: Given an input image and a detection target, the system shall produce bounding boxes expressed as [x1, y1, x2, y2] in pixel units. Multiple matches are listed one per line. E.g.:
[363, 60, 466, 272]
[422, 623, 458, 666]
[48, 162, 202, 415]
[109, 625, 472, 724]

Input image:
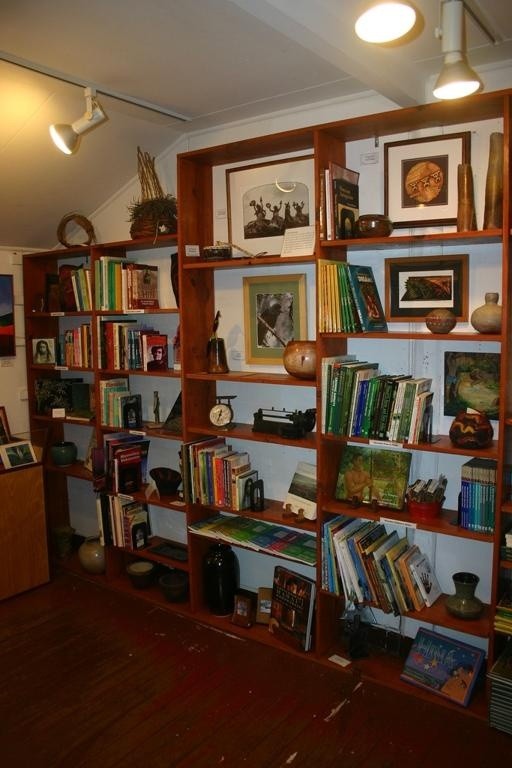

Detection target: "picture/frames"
[385, 254, 469, 322]
[0, 440, 37, 470]
[30, 337, 58, 366]
[226, 155, 314, 260]
[242, 273, 306, 366]
[384, 131, 471, 229]
[0, 406, 13, 443]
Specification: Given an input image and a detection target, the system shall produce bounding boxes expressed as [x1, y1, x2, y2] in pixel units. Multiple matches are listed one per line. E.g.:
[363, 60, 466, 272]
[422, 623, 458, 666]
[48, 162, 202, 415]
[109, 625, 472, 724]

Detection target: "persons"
[35, 341, 53, 364]
[254, 199, 304, 228]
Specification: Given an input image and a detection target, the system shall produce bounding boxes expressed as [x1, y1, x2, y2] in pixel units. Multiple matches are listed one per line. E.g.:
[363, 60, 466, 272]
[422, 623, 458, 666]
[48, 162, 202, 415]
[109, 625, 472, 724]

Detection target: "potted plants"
[125, 146, 177, 239]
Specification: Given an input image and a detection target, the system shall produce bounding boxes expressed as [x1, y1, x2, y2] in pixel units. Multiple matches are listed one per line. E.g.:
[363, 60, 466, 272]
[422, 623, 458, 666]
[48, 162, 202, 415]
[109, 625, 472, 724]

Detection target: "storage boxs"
[486, 653, 512, 735]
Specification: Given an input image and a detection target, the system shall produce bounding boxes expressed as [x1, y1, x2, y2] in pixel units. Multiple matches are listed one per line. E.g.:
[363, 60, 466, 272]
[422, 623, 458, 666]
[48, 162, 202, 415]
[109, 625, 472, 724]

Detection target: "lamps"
[431, 0, 481, 100]
[49, 87, 107, 155]
[355, 0, 416, 43]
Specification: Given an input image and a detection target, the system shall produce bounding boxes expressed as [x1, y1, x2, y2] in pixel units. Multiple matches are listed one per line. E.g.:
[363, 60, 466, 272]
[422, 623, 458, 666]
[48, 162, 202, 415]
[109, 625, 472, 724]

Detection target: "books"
[60, 378, 142, 428]
[335, 446, 500, 533]
[317, 259, 388, 333]
[97, 493, 151, 547]
[60, 319, 168, 371]
[84, 429, 150, 490]
[320, 161, 360, 240]
[487, 533, 511, 733]
[322, 355, 434, 445]
[71, 257, 160, 310]
[188, 514, 317, 651]
[320, 515, 441, 617]
[182, 438, 259, 510]
[283, 463, 317, 520]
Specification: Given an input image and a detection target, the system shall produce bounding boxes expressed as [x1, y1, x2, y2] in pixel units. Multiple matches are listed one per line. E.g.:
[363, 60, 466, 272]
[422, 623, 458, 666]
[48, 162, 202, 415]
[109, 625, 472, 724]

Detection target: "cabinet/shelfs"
[21, 236, 191, 622]
[179, 90, 512, 766]
[0, 437, 49, 600]
